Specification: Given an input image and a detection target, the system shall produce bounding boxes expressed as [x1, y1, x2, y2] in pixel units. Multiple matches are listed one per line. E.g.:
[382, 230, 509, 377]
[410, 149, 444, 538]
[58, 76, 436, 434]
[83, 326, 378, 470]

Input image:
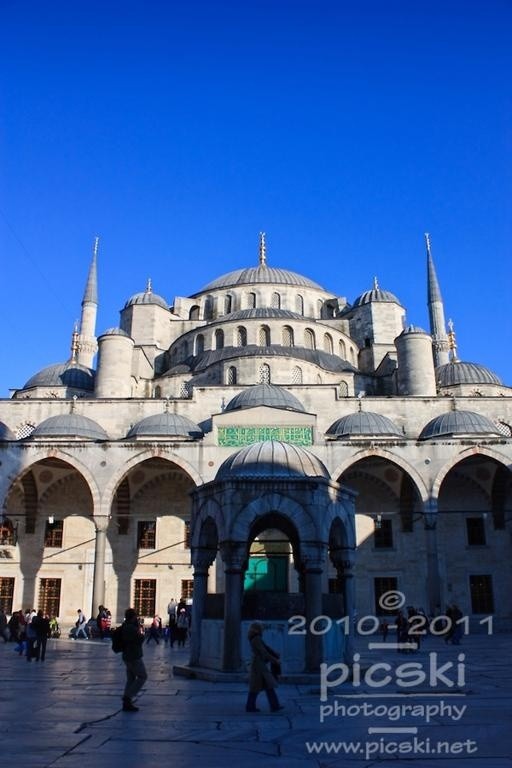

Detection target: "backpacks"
[113, 627, 125, 653]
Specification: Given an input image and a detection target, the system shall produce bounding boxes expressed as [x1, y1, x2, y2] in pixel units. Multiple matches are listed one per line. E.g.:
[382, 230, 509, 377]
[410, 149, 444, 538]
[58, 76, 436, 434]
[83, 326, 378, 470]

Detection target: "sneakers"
[245, 705, 286, 712]
[121, 696, 139, 712]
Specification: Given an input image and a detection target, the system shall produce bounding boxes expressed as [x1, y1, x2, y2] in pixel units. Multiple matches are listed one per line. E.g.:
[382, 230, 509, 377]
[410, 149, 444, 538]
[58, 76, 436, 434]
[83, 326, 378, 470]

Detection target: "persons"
[379, 604, 464, 655]
[68, 605, 112, 641]
[122, 608, 148, 712]
[137, 598, 192, 649]
[0, 608, 58, 663]
[245, 622, 286, 713]
